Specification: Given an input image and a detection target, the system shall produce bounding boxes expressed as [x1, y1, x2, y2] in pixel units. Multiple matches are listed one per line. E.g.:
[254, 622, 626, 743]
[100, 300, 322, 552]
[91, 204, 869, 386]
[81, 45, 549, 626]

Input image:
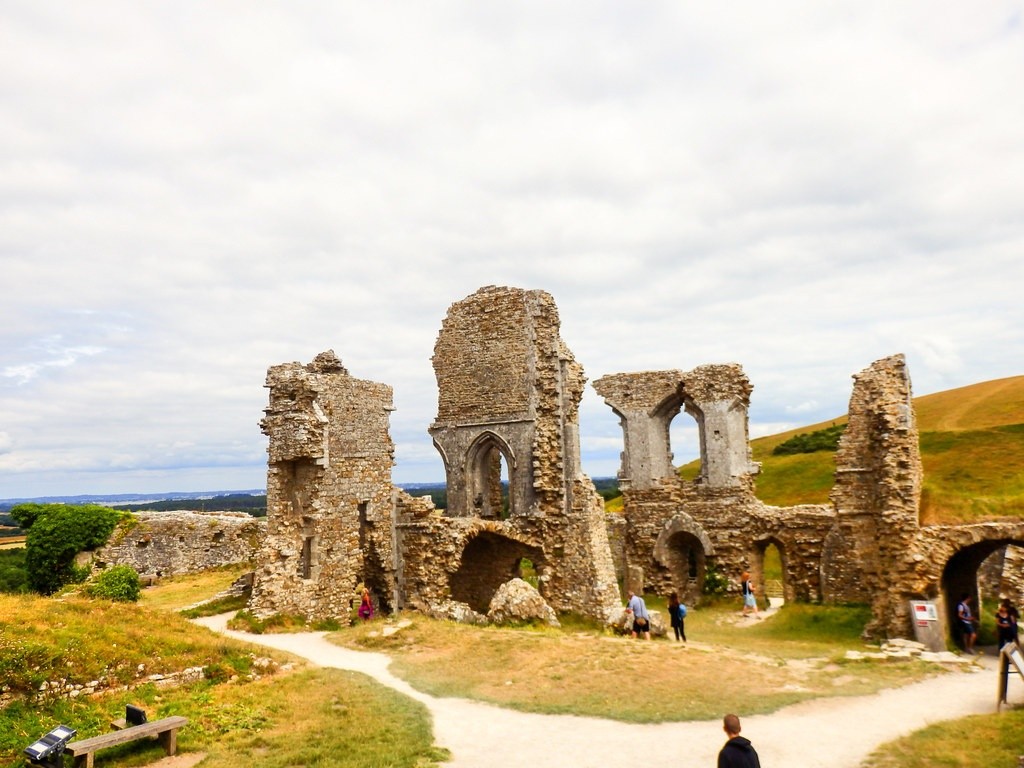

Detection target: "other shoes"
[968, 649, 975, 654]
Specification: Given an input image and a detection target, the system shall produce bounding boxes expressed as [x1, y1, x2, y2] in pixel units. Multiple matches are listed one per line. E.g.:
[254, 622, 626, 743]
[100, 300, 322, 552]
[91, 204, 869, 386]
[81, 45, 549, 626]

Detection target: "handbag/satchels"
[679, 604, 686, 618]
[637, 618, 646, 625]
[746, 593, 756, 605]
[364, 611, 370, 615]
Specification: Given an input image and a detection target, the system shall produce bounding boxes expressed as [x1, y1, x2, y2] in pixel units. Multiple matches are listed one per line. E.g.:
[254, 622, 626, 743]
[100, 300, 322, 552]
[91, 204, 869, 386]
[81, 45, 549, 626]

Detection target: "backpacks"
[954, 601, 965, 621]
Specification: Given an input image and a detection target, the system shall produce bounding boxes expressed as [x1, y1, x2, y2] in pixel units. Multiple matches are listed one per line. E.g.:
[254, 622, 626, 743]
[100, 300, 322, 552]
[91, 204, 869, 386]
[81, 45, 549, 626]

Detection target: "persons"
[358, 588, 374, 621]
[667, 592, 687, 642]
[741, 572, 761, 619]
[958, 594, 978, 654]
[995, 599, 1024, 664]
[717, 714, 761, 768]
[623, 590, 650, 640]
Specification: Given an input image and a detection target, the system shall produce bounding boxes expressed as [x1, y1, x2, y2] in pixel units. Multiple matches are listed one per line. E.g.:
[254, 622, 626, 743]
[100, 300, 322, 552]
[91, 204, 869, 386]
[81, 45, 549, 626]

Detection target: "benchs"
[64, 716, 188, 768]
